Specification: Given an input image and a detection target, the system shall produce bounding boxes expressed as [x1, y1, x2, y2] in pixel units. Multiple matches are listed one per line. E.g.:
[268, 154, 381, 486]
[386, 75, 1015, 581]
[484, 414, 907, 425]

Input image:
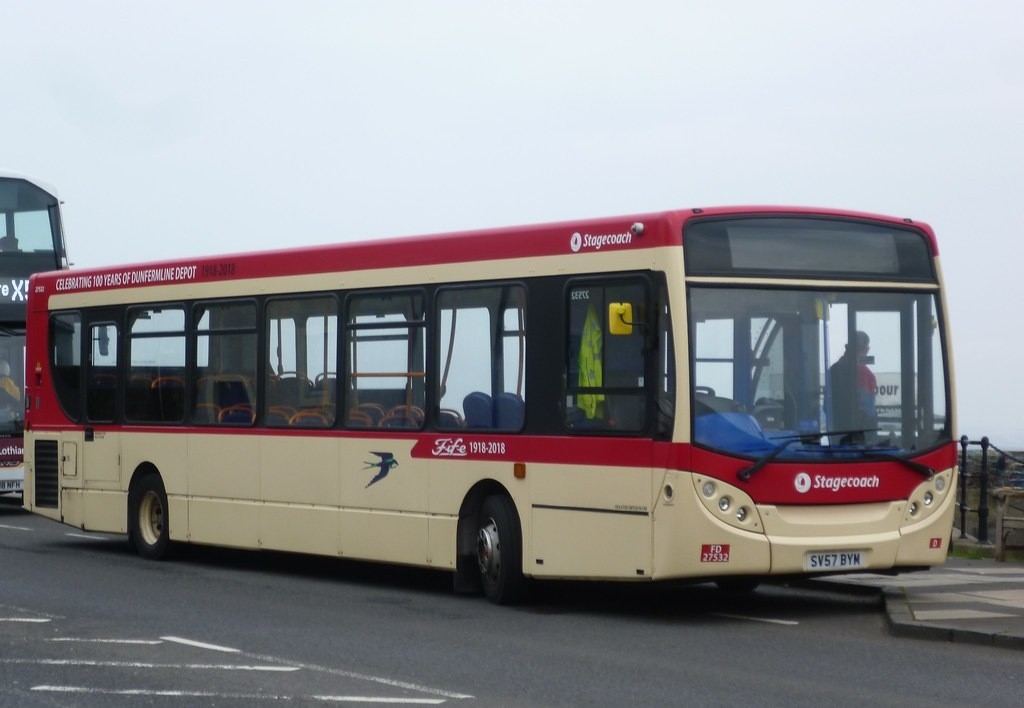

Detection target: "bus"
[0, 172, 71, 494]
[22, 207, 958, 606]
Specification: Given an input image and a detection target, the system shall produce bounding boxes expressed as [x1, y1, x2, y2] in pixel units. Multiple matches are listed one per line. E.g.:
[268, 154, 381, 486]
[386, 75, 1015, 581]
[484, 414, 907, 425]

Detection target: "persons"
[823, 331, 877, 445]
[0, 360, 20, 421]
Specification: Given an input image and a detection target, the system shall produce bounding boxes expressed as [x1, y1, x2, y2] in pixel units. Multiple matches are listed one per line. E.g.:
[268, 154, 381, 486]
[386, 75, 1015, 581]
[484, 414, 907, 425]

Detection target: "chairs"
[0, 236, 18, 250]
[91, 372, 525, 433]
